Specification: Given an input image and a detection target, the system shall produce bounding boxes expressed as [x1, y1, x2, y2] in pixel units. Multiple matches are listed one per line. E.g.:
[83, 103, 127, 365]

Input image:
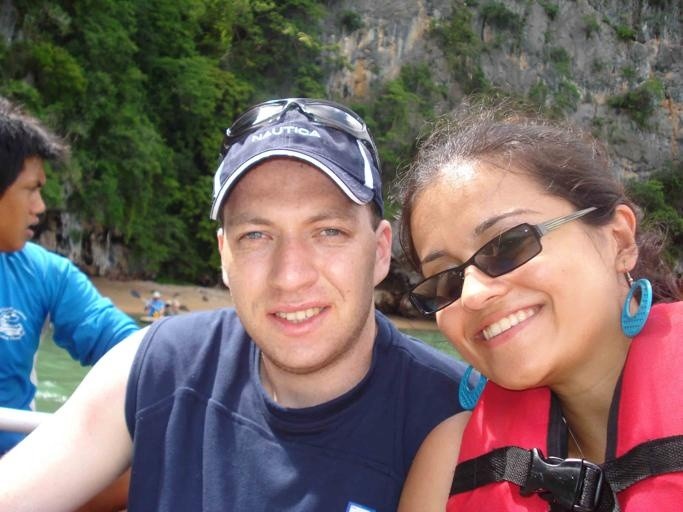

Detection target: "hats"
[209, 100, 384, 221]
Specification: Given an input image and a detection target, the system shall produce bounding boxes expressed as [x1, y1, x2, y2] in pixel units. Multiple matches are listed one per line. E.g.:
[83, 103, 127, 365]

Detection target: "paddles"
[129, 288, 148, 307]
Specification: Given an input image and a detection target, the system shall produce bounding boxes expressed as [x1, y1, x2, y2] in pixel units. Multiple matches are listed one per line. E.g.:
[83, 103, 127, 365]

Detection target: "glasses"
[409, 205, 596, 319]
[223, 98, 380, 168]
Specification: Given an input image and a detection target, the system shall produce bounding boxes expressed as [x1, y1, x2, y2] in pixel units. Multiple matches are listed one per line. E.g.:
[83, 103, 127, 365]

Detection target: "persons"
[388, 106, 682, 512]
[0, 97, 496, 512]
[141, 292, 164, 316]
[161, 300, 173, 317]
[0, 100, 142, 512]
[170, 298, 180, 316]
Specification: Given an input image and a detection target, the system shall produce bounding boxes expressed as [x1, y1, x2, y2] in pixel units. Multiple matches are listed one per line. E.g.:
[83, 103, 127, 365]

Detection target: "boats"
[138, 314, 167, 324]
